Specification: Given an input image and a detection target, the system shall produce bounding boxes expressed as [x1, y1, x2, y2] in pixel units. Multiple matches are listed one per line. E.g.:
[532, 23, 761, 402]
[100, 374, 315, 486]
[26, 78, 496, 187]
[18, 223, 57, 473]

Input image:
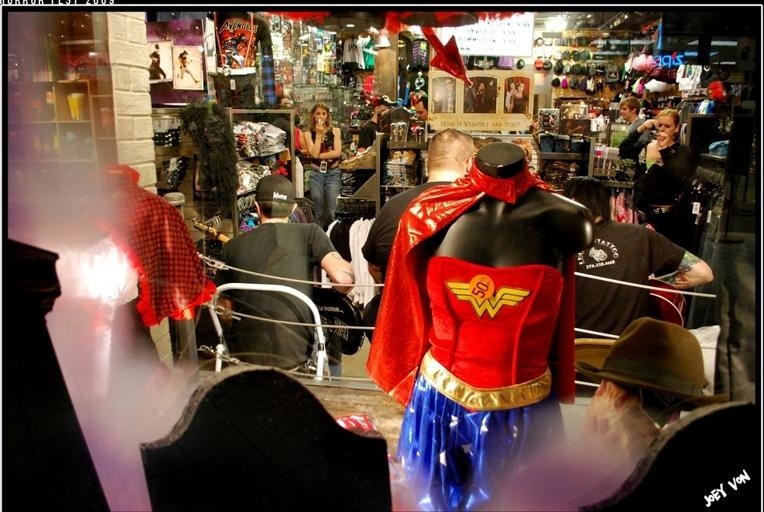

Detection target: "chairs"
[209, 283, 332, 381]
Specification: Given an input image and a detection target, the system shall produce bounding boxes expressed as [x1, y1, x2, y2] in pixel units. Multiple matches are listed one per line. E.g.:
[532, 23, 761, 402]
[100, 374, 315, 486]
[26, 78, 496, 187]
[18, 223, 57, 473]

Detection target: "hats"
[535, 38, 604, 94]
[374, 95, 396, 106]
[256, 175, 296, 203]
[575, 317, 730, 411]
[517, 59, 525, 69]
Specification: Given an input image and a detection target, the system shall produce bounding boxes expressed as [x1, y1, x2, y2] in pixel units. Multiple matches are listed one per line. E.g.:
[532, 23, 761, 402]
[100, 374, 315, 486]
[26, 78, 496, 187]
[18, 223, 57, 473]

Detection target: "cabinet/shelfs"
[9, 12, 112, 233]
[376, 131, 429, 218]
[203, 107, 297, 238]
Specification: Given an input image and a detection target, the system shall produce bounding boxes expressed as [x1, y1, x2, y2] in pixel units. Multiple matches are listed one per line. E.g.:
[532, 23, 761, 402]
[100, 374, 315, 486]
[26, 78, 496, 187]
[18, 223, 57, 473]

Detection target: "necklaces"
[655, 156, 662, 164]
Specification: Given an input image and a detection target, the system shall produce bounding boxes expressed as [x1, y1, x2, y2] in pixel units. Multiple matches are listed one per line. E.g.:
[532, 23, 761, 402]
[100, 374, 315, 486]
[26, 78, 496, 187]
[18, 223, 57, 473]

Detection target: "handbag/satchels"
[606, 64, 619, 83]
[622, 55, 667, 98]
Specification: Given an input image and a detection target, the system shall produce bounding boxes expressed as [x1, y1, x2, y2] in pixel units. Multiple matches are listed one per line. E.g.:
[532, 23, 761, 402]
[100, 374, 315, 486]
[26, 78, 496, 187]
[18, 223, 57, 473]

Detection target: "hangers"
[689, 166, 727, 208]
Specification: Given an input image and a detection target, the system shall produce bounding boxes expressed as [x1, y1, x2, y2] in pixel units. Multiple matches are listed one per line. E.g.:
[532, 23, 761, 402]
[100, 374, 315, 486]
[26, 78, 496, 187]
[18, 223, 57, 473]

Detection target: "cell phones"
[320, 161, 327, 174]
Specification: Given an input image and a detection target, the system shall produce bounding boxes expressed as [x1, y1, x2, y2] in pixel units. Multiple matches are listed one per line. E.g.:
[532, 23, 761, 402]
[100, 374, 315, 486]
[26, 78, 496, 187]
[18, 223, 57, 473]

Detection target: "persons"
[509, 83, 528, 114]
[364, 142, 594, 512]
[361, 129, 476, 292]
[433, 78, 455, 113]
[563, 174, 714, 340]
[465, 84, 476, 113]
[293, 113, 308, 199]
[176, 51, 200, 84]
[620, 96, 650, 144]
[303, 102, 342, 228]
[277, 95, 291, 108]
[415, 96, 428, 122]
[148, 44, 166, 80]
[618, 108, 693, 250]
[357, 96, 398, 152]
[213, 175, 356, 370]
[505, 80, 517, 112]
[576, 318, 730, 461]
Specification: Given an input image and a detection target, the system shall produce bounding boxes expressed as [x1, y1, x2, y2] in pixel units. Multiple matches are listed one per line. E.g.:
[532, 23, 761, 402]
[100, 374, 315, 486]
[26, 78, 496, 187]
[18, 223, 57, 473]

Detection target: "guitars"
[646, 275, 689, 328]
[192, 216, 365, 355]
